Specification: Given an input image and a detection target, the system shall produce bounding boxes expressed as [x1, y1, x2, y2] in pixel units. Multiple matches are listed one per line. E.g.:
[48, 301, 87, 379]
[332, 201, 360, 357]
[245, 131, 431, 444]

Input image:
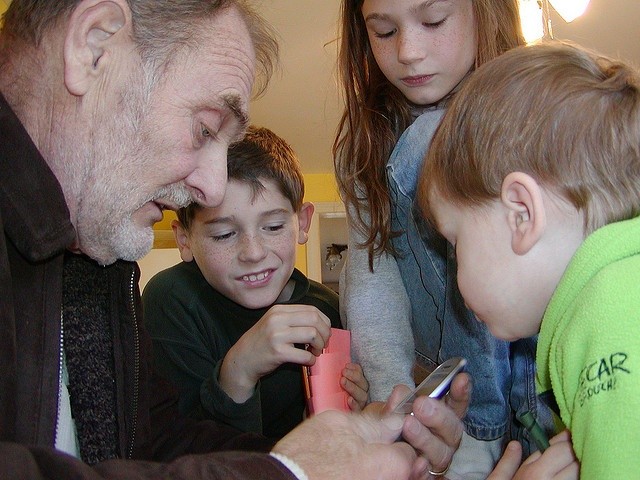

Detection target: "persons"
[331, 2, 555, 480]
[416, 42, 638, 480]
[1, 1, 472, 480]
[141, 127, 369, 433]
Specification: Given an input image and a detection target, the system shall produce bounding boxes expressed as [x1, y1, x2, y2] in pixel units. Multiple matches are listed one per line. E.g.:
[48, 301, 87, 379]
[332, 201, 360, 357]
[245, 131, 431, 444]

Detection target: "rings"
[427, 463, 452, 477]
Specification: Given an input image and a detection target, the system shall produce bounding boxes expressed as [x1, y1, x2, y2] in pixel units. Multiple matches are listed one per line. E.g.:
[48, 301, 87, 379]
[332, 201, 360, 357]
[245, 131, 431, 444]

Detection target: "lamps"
[325, 243, 349, 271]
[516, 0, 592, 46]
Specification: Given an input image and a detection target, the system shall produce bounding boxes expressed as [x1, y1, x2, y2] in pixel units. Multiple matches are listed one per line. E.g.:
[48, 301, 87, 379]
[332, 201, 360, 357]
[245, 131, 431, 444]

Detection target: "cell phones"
[391, 357, 467, 443]
